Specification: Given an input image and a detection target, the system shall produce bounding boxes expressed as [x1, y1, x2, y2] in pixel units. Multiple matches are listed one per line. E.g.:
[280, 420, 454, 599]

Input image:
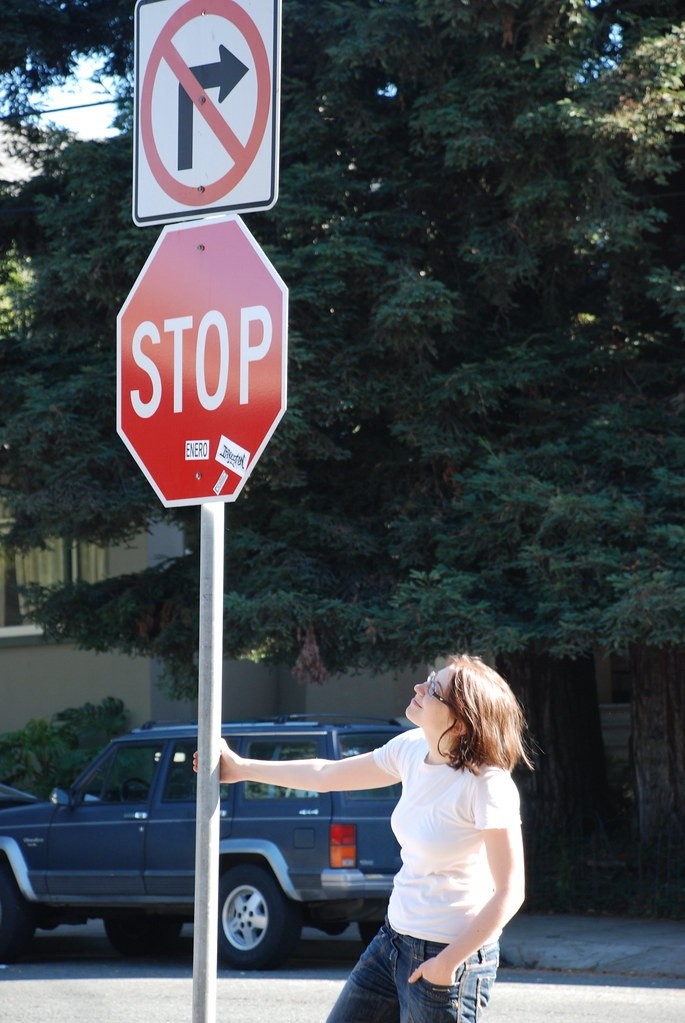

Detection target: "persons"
[193, 656, 534, 1023]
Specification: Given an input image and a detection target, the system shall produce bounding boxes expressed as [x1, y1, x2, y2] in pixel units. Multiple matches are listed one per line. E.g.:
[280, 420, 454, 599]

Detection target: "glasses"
[427, 670, 453, 709]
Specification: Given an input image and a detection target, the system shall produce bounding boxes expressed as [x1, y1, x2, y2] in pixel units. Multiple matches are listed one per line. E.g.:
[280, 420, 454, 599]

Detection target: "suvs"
[0, 713, 419, 970]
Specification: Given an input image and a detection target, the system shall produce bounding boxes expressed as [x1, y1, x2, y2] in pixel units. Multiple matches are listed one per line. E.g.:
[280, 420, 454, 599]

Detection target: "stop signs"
[115, 212, 290, 508]
